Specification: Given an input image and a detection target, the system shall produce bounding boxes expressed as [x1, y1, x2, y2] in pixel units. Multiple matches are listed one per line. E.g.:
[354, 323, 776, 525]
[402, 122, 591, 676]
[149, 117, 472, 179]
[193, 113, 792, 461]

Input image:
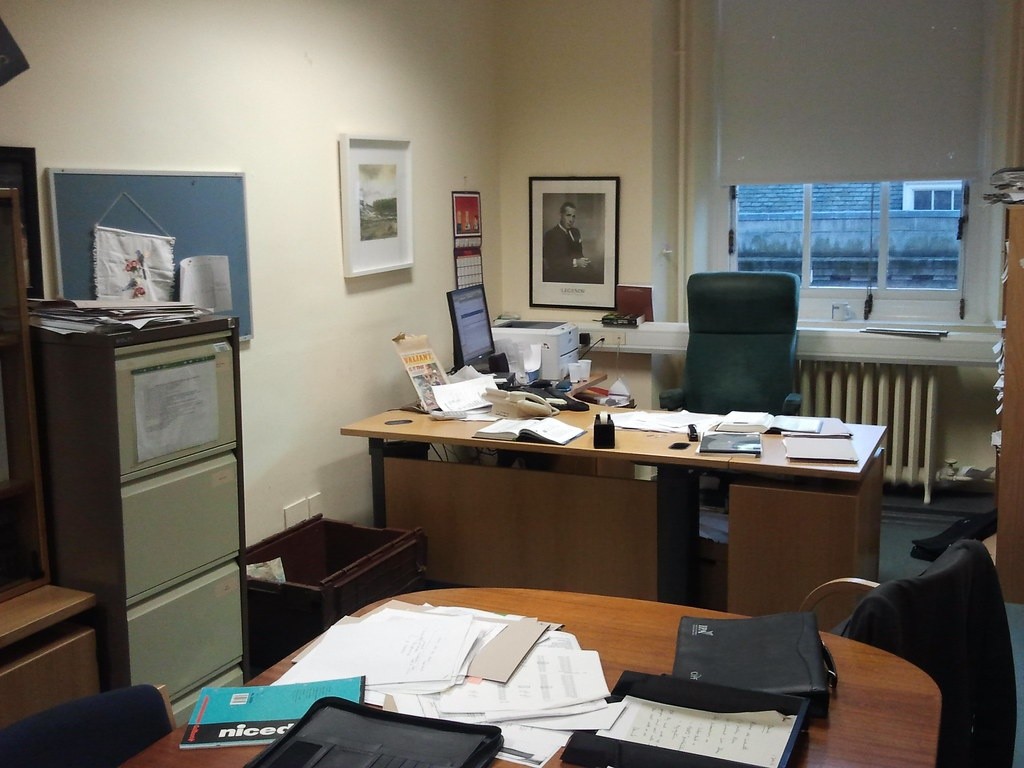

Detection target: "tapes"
[600, 411, 608, 424]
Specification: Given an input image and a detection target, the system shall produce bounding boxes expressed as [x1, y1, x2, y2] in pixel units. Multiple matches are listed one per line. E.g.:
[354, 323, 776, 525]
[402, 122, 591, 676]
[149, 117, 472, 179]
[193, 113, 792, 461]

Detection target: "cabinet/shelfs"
[35, 314, 251, 720]
[994, 203, 1024, 603]
[0, 186, 106, 731]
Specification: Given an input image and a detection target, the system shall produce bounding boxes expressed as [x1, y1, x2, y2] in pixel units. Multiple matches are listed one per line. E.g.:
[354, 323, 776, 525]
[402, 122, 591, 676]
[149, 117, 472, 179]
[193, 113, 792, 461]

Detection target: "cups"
[568, 363, 582, 382]
[832, 303, 851, 321]
[577, 360, 592, 379]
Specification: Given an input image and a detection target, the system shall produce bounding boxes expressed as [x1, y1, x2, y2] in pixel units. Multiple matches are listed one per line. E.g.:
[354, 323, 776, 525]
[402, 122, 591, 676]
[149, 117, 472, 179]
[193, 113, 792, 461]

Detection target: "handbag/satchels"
[672, 610, 838, 721]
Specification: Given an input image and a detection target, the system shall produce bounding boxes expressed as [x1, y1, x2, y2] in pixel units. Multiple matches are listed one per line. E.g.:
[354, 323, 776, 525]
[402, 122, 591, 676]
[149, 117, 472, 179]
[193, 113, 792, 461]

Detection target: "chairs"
[0, 682, 178, 768]
[658, 270, 805, 415]
[798, 539, 985, 681]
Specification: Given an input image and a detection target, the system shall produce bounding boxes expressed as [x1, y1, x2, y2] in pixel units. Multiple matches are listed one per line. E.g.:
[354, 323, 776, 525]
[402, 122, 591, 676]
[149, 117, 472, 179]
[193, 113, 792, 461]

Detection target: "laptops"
[517, 388, 576, 410]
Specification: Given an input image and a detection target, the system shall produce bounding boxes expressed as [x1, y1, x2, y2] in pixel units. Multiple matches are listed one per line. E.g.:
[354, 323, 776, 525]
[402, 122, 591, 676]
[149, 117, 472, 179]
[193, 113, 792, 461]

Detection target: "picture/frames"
[339, 131, 414, 279]
[527, 176, 620, 311]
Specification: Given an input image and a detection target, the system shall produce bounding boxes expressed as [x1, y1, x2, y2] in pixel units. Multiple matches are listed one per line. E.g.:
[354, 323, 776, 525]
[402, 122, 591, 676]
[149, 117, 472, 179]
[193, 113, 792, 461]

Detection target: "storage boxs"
[246, 513, 426, 656]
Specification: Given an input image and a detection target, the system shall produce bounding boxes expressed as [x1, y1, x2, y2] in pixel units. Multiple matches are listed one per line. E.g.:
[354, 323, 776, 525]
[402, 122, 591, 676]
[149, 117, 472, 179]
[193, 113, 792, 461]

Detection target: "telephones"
[482, 387, 553, 420]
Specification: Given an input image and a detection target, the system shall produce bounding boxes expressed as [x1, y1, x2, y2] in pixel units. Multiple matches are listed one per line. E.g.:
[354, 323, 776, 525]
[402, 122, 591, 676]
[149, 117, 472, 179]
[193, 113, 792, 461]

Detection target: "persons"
[544, 200, 593, 283]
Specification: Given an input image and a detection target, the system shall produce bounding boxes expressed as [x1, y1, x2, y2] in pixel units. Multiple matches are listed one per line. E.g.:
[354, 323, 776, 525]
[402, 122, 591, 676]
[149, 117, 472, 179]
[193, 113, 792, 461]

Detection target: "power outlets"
[578, 331, 626, 345]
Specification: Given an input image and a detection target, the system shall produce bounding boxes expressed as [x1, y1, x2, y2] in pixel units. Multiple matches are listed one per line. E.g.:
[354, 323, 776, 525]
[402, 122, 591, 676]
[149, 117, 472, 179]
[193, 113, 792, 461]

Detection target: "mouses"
[529, 379, 553, 388]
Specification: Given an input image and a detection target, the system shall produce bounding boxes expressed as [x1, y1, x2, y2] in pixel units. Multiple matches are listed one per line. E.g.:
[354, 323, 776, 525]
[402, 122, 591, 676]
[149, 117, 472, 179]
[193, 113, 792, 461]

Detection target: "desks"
[338, 370, 887, 639]
[120, 586, 946, 768]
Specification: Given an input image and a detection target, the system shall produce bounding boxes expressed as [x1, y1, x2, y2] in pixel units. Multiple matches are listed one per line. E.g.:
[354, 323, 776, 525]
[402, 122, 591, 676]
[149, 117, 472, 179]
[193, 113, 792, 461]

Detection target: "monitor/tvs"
[446, 283, 496, 373]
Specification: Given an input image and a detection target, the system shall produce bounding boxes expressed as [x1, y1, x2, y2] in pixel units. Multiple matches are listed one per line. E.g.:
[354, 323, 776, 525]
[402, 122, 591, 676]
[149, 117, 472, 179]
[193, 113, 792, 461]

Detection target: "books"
[178, 676, 366, 750]
[699, 411, 859, 464]
[602, 312, 646, 326]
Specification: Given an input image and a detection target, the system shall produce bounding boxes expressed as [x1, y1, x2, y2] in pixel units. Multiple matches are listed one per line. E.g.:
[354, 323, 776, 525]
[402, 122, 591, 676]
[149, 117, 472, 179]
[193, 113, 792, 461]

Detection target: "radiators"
[797, 361, 958, 506]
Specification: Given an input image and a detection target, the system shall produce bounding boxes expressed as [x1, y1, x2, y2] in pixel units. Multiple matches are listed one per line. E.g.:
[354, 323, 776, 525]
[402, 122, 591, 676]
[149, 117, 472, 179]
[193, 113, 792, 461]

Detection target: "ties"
[567, 230, 572, 241]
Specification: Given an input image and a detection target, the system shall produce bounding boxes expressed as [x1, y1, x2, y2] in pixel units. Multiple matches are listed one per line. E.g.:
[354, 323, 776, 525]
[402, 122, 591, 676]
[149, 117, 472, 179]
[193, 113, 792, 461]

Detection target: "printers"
[490, 319, 580, 386]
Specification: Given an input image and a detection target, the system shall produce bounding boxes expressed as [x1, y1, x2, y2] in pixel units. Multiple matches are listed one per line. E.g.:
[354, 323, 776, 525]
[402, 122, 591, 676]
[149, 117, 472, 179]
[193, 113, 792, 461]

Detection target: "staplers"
[687, 424, 698, 441]
